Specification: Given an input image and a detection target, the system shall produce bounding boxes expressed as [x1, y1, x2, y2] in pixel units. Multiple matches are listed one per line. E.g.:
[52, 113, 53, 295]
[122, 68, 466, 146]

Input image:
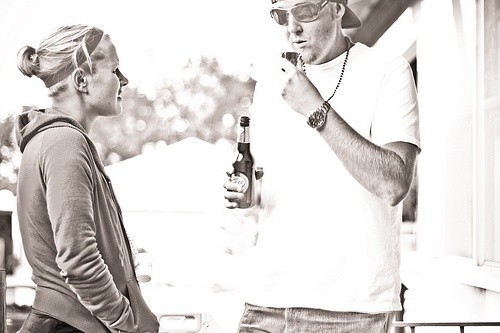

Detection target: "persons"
[14, 25, 159, 333]
[223, 0, 421, 333]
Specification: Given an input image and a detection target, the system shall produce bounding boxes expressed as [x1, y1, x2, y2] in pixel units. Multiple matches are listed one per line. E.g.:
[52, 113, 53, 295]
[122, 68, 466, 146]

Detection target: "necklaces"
[298, 35, 351, 102]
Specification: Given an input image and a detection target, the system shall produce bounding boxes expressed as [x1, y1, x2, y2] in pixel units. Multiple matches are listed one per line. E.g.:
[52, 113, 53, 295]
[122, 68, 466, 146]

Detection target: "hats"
[318, 0, 362, 28]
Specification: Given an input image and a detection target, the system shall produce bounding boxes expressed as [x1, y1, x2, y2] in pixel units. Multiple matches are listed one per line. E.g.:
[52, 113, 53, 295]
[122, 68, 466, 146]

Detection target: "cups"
[282, 52, 300, 73]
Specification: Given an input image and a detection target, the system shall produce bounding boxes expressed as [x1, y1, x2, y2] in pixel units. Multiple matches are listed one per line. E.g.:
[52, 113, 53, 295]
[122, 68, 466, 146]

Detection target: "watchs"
[306, 99, 332, 130]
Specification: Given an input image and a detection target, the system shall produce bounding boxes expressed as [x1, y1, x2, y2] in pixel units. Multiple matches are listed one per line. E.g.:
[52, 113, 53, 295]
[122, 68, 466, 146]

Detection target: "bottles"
[227, 116, 256, 208]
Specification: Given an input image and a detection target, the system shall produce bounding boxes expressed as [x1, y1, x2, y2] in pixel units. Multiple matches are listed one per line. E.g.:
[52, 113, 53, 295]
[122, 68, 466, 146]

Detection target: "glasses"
[269, 0, 328, 26]
